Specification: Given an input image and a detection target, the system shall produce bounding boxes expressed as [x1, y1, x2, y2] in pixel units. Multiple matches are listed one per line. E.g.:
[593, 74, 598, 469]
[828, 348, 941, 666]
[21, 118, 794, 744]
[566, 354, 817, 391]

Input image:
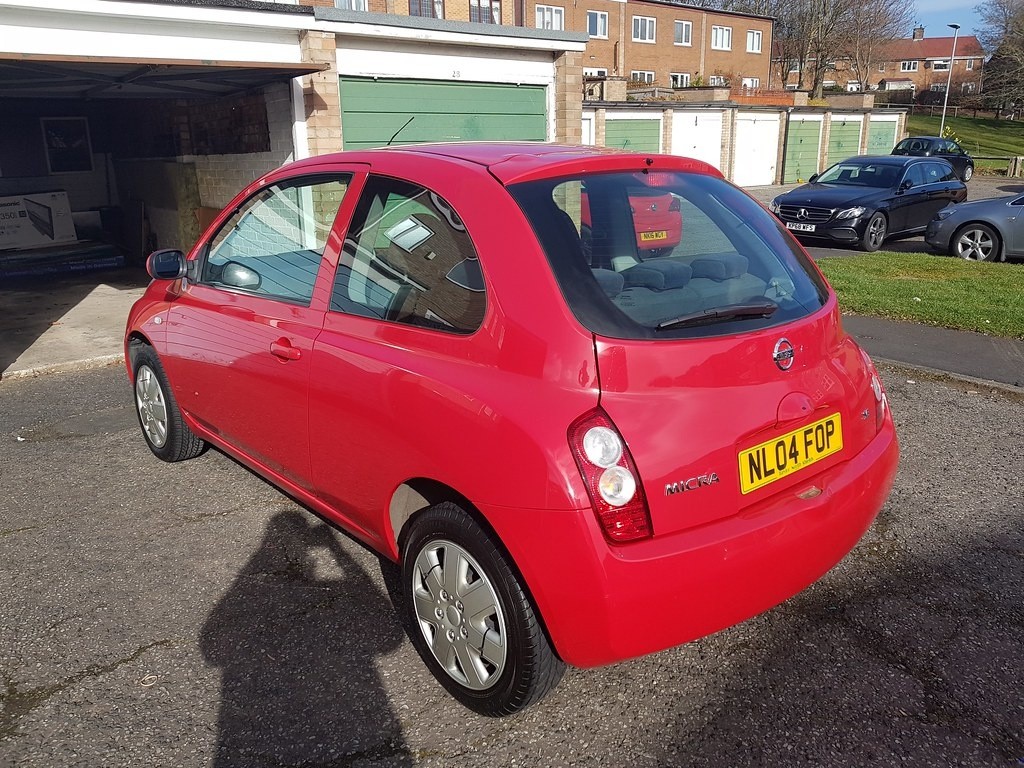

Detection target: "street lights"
[938, 23, 961, 140]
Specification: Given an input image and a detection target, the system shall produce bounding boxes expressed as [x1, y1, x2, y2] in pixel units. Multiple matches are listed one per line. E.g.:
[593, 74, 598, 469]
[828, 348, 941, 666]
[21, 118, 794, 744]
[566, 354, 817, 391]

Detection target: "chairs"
[370, 213, 452, 323]
[591, 252, 765, 323]
[858, 169, 868, 182]
[881, 167, 897, 183]
[913, 142, 920, 149]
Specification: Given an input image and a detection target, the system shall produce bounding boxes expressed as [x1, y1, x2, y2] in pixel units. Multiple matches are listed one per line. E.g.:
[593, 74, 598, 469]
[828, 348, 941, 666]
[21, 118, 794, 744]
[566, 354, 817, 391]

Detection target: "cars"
[891, 136, 975, 183]
[766, 155, 968, 252]
[120, 139, 900, 720]
[924, 193, 1024, 264]
